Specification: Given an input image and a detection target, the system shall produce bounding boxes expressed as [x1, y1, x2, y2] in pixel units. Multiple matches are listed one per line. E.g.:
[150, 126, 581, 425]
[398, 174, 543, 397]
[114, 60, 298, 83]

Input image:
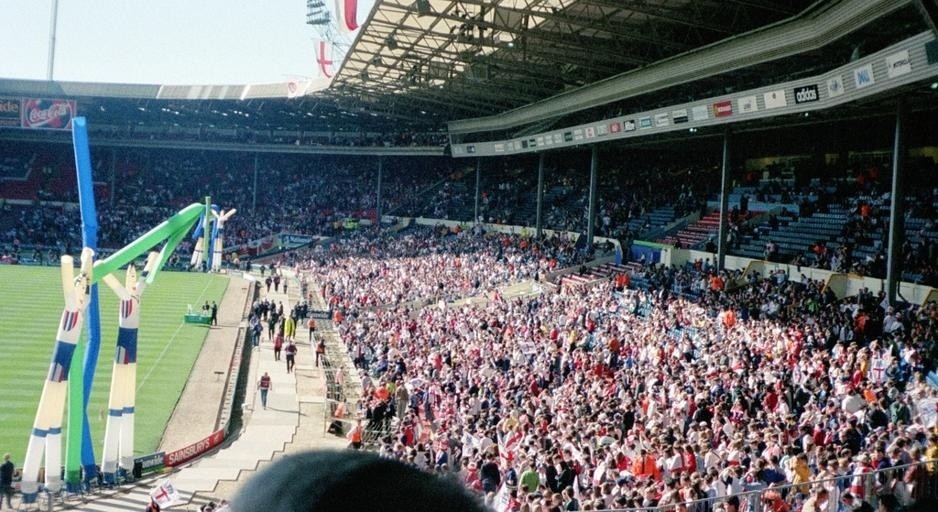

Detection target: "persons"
[1, 89, 938, 512]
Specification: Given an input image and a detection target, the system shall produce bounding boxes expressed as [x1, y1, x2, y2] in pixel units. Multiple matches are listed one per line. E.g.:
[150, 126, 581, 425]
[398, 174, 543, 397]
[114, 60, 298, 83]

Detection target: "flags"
[334, 0, 359, 33]
[311, 37, 333, 79]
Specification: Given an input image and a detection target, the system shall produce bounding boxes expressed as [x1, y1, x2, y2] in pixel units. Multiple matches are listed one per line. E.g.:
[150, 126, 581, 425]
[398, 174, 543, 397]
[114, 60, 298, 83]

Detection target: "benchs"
[558, 163, 936, 347]
